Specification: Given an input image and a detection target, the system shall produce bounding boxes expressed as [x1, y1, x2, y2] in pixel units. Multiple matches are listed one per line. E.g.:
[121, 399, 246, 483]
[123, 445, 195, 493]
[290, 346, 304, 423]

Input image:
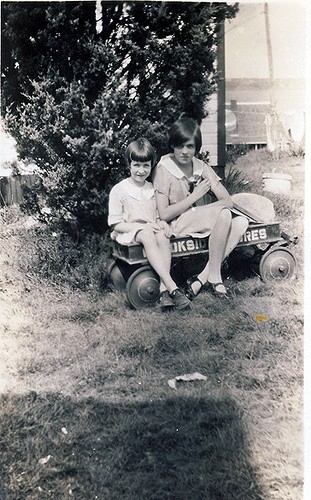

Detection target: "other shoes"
[171, 289, 190, 308]
[161, 290, 175, 308]
[184, 274, 204, 301]
[206, 280, 234, 303]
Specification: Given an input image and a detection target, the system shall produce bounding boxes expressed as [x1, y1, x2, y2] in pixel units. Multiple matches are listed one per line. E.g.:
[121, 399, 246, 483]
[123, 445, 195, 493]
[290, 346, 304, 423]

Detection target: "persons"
[150, 119, 249, 300]
[109, 137, 191, 309]
